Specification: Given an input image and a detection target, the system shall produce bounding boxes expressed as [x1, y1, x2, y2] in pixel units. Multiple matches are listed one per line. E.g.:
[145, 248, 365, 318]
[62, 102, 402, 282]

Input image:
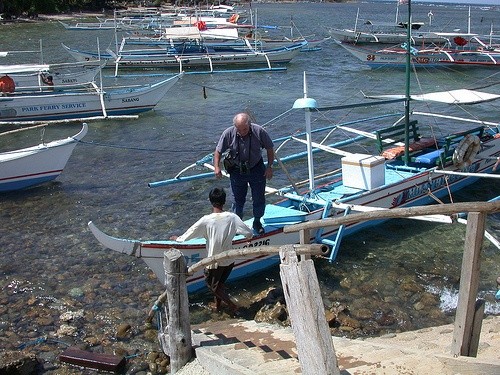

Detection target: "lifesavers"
[453, 134, 480, 168]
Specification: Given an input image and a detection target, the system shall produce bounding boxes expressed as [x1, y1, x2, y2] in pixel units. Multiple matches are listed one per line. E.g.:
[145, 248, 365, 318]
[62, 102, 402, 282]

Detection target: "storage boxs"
[341, 154, 385, 190]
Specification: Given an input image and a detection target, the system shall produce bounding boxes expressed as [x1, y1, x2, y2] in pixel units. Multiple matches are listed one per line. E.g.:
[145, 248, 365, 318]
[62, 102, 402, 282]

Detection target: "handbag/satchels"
[223, 147, 239, 173]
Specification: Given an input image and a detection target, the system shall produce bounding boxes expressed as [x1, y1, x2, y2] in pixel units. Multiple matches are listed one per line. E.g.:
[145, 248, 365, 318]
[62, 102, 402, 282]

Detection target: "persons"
[169, 188, 254, 318]
[213, 113, 275, 236]
[40, 73, 55, 90]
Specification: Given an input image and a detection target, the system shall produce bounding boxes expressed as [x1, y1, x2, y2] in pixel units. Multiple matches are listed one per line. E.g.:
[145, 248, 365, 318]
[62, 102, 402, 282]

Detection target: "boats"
[329, 0, 500, 69]
[87, 0, 500, 295]
[0, 0, 331, 196]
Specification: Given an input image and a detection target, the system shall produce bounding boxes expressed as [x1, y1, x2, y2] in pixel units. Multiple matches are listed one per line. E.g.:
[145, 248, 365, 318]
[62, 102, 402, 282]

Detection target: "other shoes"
[253, 221, 265, 233]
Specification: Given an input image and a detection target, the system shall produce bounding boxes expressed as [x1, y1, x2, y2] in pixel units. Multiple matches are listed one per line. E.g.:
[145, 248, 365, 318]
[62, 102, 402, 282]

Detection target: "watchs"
[266, 164, 273, 167]
